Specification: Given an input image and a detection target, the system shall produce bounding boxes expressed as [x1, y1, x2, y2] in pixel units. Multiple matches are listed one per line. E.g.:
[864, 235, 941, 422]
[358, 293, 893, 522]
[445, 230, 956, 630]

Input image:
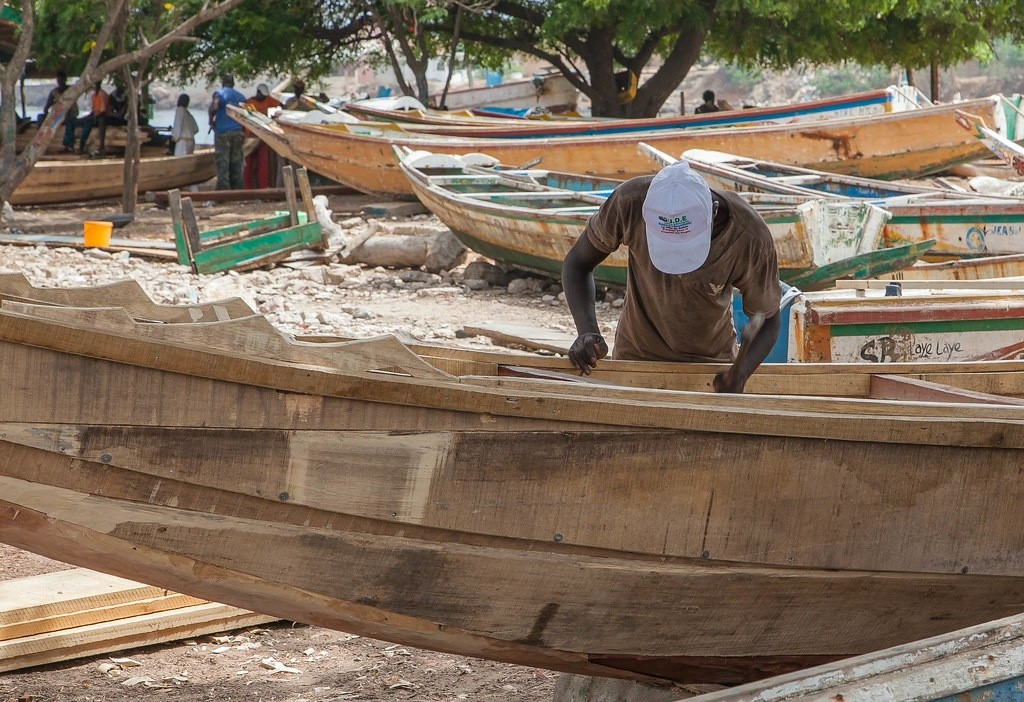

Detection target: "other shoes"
[56, 147, 85, 155]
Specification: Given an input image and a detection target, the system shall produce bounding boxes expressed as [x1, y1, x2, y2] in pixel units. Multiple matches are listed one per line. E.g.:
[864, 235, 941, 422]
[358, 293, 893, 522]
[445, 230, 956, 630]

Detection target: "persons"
[88, 76, 131, 159]
[208, 73, 247, 191]
[694, 91, 720, 114]
[36, 71, 78, 152]
[285, 77, 316, 110]
[59, 79, 113, 154]
[241, 82, 286, 190]
[558, 161, 784, 396]
[170, 94, 200, 155]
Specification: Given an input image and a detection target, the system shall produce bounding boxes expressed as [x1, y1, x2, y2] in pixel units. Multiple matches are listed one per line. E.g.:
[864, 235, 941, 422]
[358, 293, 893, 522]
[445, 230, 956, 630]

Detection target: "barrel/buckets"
[83, 219, 113, 248]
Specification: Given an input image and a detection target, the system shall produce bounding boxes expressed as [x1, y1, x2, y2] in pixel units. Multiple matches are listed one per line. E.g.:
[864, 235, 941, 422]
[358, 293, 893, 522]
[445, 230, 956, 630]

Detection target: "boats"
[1, 67, 1023, 702]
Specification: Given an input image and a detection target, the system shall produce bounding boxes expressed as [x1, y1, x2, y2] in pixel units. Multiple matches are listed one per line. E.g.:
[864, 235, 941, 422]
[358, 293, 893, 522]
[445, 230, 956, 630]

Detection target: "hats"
[257, 84, 269, 96]
[642, 161, 713, 274]
